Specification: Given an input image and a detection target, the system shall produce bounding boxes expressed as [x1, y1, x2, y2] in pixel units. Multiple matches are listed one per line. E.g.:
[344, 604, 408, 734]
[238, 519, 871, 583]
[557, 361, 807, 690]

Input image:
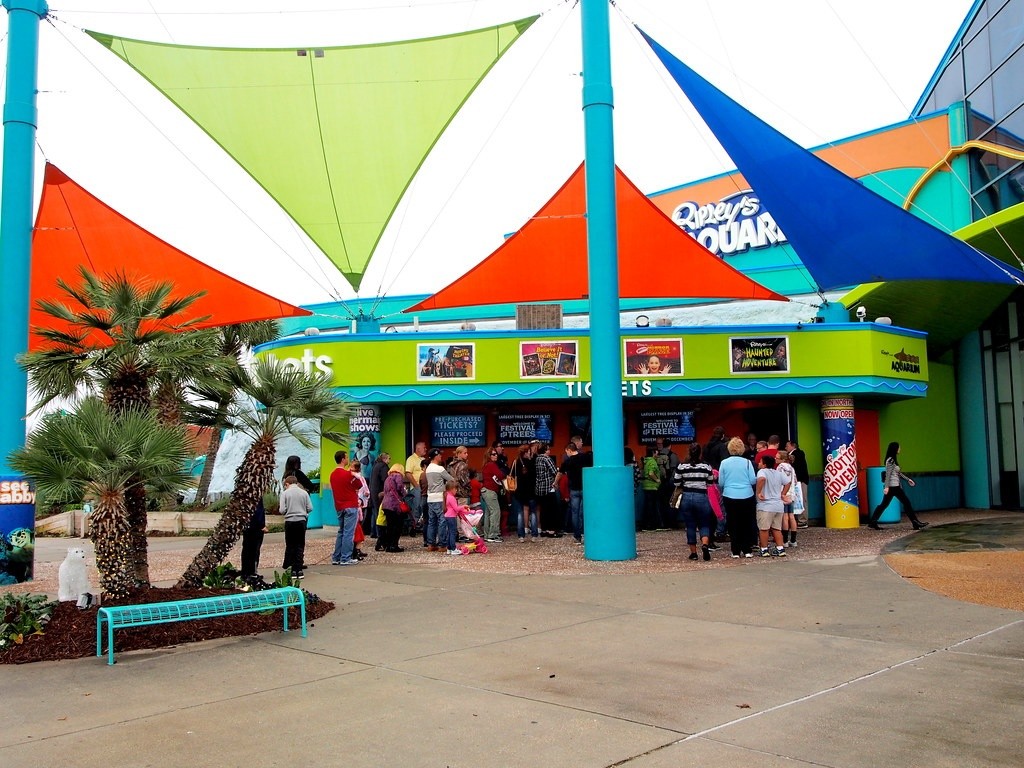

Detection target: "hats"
[429, 448, 444, 457]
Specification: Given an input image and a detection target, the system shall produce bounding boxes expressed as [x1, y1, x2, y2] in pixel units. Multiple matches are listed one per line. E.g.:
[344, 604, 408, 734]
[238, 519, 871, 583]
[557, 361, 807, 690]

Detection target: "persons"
[638, 356, 672, 375]
[674, 426, 809, 561]
[350, 433, 375, 488]
[242, 496, 268, 582]
[329, 436, 593, 565]
[282, 455, 313, 569]
[423, 348, 463, 378]
[279, 477, 312, 579]
[774, 346, 786, 370]
[866, 442, 930, 530]
[624, 436, 679, 532]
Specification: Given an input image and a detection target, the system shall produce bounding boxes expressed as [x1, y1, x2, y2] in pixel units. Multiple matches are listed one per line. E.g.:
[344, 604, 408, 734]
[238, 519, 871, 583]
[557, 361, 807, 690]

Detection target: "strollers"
[457, 508, 488, 555]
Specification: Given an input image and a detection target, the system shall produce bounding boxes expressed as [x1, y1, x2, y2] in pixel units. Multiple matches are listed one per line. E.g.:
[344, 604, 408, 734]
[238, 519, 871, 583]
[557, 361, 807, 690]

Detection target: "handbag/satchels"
[669, 485, 683, 509]
[506, 460, 517, 491]
[881, 471, 886, 483]
[400, 501, 410, 513]
[793, 482, 805, 514]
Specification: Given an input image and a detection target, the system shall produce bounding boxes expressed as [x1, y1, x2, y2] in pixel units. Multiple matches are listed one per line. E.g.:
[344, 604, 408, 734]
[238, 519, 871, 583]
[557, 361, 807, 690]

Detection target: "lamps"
[635, 315, 650, 327]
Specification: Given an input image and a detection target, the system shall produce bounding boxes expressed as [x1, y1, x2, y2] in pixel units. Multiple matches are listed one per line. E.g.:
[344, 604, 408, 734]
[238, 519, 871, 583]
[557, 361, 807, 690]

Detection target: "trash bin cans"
[307, 479, 323, 530]
[867, 466, 901, 524]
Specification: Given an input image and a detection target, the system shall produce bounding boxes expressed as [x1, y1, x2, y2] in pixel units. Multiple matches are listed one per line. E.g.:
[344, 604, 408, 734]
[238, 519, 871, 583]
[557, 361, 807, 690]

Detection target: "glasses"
[490, 453, 497, 455]
[775, 457, 780, 460]
[756, 446, 763, 450]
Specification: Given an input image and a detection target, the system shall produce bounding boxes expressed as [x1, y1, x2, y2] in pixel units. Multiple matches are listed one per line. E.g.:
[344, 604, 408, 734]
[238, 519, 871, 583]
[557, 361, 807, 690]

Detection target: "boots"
[868, 505, 885, 530]
[904, 507, 929, 529]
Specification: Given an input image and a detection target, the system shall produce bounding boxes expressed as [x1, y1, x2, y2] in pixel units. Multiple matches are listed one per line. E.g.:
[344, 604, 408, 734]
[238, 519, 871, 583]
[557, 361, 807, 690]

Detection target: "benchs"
[97, 586, 309, 666]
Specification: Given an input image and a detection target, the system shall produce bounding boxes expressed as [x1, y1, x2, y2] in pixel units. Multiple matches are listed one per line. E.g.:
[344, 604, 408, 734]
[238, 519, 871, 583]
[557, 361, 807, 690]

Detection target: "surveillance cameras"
[857, 306, 866, 316]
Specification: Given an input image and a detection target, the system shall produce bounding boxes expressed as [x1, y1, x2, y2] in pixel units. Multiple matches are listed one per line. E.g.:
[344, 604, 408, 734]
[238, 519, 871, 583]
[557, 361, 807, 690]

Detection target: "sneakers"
[332, 560, 340, 564]
[428, 528, 584, 556]
[340, 559, 358, 566]
[643, 519, 811, 562]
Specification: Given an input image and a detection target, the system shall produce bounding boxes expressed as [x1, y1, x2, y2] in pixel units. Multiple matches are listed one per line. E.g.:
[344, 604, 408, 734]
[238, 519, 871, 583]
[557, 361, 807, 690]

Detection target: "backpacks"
[656, 451, 673, 481]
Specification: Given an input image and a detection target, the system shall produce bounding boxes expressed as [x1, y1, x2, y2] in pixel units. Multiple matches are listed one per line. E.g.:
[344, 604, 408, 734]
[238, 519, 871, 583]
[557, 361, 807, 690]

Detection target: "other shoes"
[358, 549, 367, 557]
[291, 573, 304, 579]
[375, 545, 380, 550]
[351, 553, 363, 560]
[386, 547, 404, 553]
[302, 565, 307, 569]
